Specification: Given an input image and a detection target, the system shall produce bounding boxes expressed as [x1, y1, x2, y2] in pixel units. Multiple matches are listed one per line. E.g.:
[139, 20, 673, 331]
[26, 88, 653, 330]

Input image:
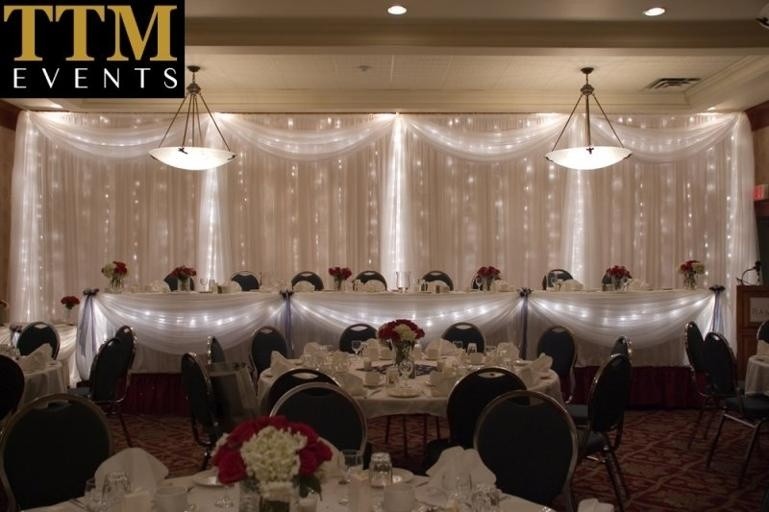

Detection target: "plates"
[540, 371, 552, 378]
[287, 357, 303, 365]
[365, 467, 414, 486]
[516, 360, 533, 365]
[192, 469, 225, 488]
[387, 388, 420, 398]
[264, 370, 272, 376]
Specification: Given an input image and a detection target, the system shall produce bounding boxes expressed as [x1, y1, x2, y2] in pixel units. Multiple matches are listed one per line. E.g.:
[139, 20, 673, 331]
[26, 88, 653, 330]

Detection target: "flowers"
[214, 416, 334, 498]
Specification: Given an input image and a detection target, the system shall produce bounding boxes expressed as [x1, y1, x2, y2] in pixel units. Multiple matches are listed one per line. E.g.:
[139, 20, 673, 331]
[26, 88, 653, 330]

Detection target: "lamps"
[149, 65, 238, 172]
[543, 66, 634, 172]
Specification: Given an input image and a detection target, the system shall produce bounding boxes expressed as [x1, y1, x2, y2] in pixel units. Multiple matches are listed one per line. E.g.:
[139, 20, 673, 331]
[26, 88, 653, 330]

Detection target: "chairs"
[267, 383, 368, 468]
[0, 258, 769, 448]
[568, 352, 632, 509]
[702, 332, 769, 488]
[475, 393, 579, 508]
[2, 391, 112, 511]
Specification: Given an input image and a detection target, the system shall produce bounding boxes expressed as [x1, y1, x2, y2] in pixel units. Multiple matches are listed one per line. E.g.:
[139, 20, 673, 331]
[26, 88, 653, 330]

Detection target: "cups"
[237, 482, 258, 511]
[383, 481, 417, 511]
[368, 453, 393, 488]
[213, 484, 236, 509]
[449, 472, 498, 512]
[297, 494, 319, 512]
[85, 470, 200, 512]
[305, 341, 515, 387]
[127, 272, 689, 293]
[346, 478, 370, 511]
[338, 449, 364, 481]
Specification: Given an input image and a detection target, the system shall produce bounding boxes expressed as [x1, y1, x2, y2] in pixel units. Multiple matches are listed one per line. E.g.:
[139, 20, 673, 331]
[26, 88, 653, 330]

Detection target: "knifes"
[364, 389, 383, 398]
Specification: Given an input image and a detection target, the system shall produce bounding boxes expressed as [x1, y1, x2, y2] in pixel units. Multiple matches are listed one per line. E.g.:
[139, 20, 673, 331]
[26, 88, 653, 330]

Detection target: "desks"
[48, 466, 558, 512]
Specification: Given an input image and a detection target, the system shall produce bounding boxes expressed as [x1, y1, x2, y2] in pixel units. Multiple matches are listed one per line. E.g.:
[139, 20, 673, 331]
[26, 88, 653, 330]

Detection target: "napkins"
[95, 448, 167, 492]
[426, 447, 497, 490]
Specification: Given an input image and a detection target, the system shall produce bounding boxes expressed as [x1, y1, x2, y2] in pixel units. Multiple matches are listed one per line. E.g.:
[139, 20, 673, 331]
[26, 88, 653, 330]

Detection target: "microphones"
[755, 260, 761, 281]
